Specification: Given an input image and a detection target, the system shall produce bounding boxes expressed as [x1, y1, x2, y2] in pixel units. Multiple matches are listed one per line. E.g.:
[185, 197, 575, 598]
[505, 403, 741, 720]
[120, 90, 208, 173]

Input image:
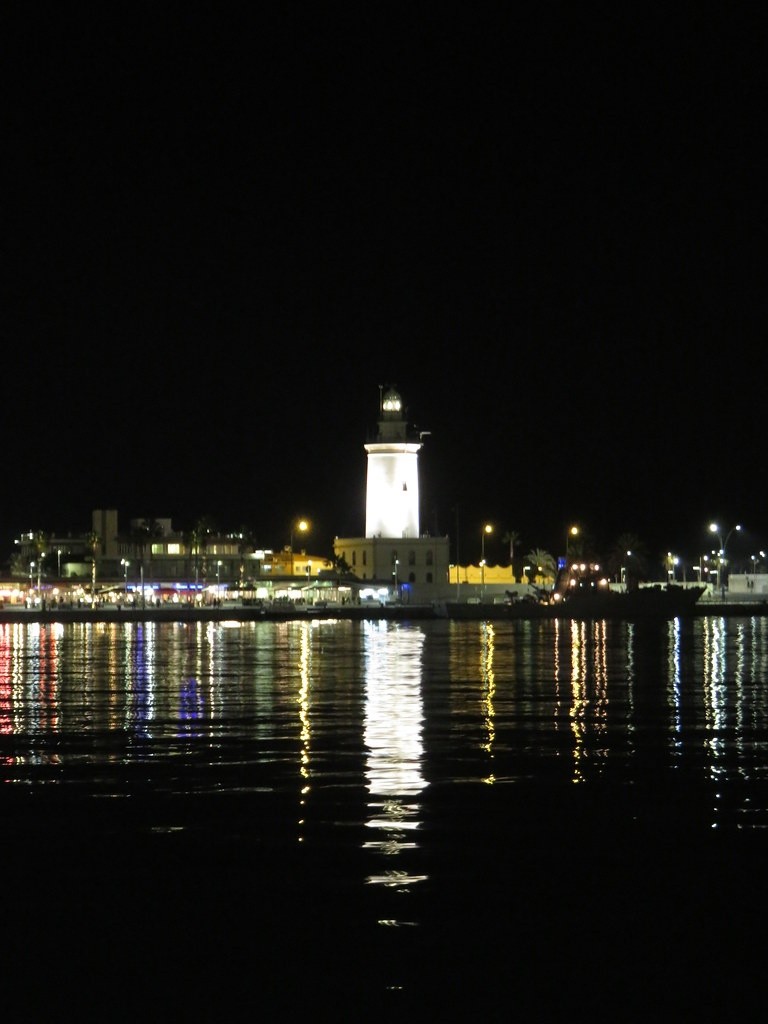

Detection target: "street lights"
[218, 561, 222, 597]
[308, 560, 312, 586]
[710, 521, 742, 603]
[395, 560, 399, 590]
[481, 525, 492, 585]
[120, 559, 130, 597]
[291, 521, 308, 552]
[667, 552, 675, 586]
[57, 550, 62, 581]
[30, 562, 35, 590]
[566, 527, 578, 566]
[38, 552, 45, 592]
